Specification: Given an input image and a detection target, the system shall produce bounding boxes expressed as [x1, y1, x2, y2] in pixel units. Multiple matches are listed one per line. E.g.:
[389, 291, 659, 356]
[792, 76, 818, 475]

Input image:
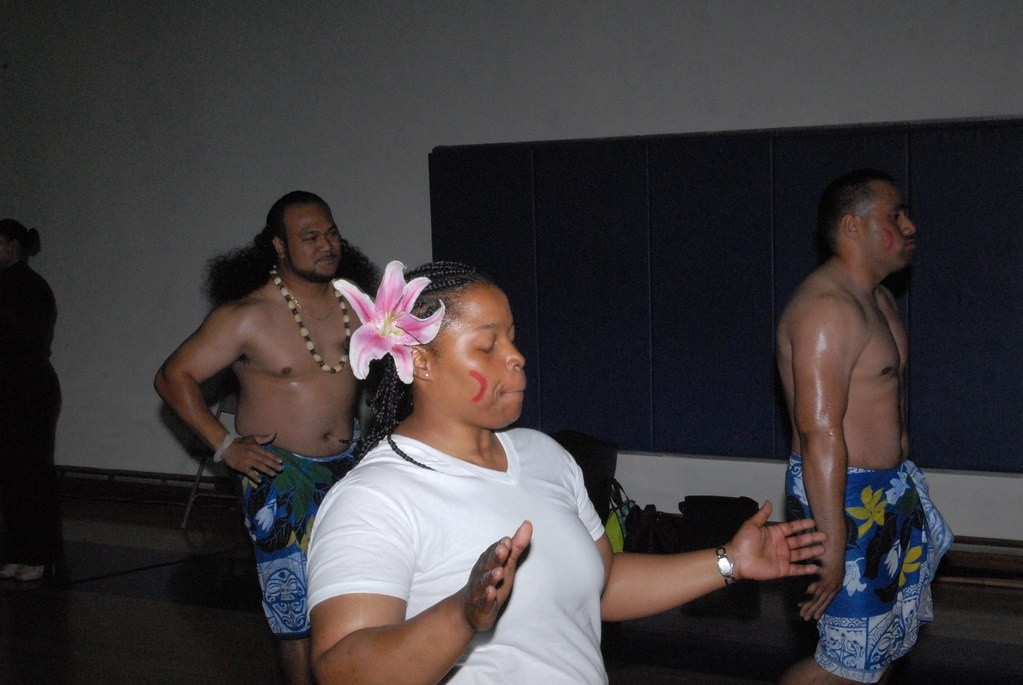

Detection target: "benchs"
[935, 535, 1023, 591]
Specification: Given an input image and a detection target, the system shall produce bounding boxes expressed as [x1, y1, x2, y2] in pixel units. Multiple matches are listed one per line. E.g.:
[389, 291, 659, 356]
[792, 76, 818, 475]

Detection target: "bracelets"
[716, 545, 737, 585]
[213, 433, 241, 462]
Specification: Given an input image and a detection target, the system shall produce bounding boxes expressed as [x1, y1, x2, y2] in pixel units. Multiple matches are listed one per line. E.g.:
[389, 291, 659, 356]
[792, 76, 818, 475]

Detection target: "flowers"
[330, 260, 446, 384]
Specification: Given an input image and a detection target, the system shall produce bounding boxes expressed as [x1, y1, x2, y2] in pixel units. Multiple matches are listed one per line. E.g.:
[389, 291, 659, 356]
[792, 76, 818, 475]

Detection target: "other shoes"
[15, 565, 44, 580]
[0, 564, 21, 580]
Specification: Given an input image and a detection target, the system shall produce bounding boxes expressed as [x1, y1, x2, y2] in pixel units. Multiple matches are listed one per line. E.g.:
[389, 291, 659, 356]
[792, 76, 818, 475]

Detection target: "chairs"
[178, 376, 240, 528]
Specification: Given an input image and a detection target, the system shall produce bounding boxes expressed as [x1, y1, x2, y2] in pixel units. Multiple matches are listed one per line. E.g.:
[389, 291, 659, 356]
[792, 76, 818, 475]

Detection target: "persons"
[308, 256, 828, 685]
[0, 219, 62, 581]
[154, 190, 379, 685]
[777, 168, 955, 685]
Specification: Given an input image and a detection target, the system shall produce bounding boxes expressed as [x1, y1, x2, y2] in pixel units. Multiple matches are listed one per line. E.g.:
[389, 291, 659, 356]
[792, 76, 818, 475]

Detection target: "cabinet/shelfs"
[428, 112, 1023, 477]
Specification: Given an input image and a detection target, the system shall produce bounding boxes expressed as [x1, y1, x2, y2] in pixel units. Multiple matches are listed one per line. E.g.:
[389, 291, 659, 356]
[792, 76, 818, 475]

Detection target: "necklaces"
[273, 277, 351, 374]
